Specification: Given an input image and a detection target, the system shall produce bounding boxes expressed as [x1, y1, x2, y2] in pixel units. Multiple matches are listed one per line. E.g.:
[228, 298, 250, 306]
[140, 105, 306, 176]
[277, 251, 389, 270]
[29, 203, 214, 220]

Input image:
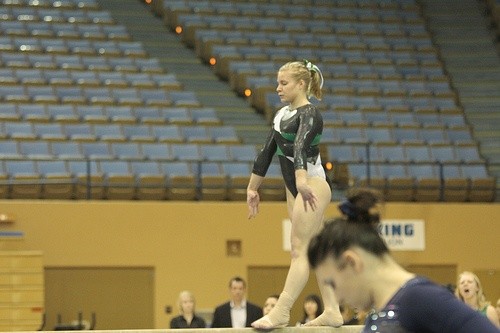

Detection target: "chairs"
[0, 0, 495, 203]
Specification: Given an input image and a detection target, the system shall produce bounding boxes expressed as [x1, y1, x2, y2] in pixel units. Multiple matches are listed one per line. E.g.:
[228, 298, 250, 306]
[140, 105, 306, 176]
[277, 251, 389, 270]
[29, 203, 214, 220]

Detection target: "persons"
[246, 59, 344, 330]
[306, 188, 500, 333]
[170, 271, 375, 333]
[453, 271, 500, 329]
